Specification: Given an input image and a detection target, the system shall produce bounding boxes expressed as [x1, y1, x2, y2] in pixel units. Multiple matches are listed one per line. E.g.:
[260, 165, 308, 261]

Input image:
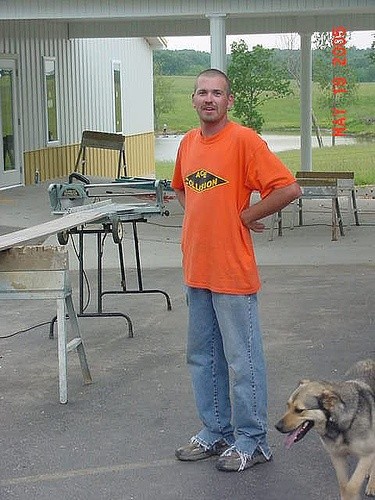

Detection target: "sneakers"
[216, 448, 266, 471]
[175, 440, 216, 460]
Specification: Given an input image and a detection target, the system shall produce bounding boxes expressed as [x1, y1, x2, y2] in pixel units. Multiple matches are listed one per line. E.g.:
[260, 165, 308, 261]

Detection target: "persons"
[169, 69, 302, 472]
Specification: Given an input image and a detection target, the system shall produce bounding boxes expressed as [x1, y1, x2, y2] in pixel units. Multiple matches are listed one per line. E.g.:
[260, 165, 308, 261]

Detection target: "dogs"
[273, 359, 375, 500]
